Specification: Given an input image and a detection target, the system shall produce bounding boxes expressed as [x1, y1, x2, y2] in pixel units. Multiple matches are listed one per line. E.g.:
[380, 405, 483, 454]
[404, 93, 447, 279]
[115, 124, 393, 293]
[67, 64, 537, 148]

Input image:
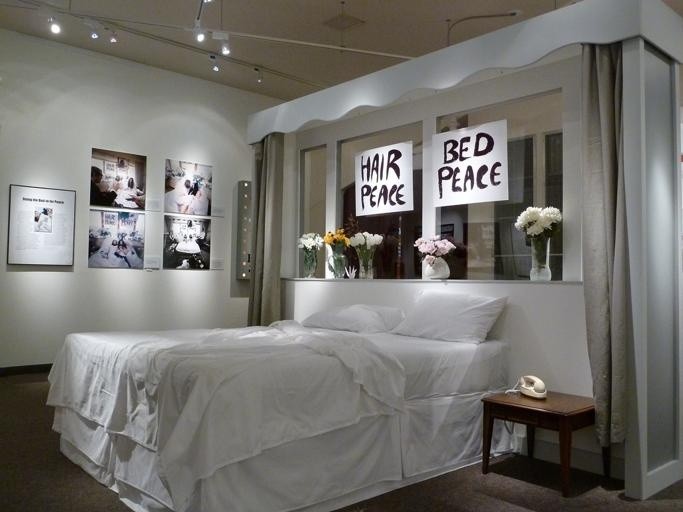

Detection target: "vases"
[326, 245, 347, 280]
[422, 254, 451, 279]
[528, 236, 553, 282]
[353, 244, 376, 280]
[302, 248, 318, 280]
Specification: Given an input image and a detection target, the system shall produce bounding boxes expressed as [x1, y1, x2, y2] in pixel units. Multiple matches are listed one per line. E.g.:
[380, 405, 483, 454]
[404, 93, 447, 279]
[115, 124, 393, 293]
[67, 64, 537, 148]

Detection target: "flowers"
[512, 204, 561, 269]
[295, 231, 323, 271]
[348, 230, 382, 274]
[413, 234, 455, 266]
[323, 228, 349, 272]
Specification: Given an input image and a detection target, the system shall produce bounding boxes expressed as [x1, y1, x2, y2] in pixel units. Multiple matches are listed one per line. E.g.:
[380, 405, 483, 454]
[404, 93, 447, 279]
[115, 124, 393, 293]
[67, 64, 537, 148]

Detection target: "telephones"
[518, 374, 548, 400]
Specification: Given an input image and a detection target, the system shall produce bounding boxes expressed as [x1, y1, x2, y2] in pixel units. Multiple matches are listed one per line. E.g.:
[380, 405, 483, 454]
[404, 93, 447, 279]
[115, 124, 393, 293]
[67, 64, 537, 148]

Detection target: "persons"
[88, 166, 206, 268]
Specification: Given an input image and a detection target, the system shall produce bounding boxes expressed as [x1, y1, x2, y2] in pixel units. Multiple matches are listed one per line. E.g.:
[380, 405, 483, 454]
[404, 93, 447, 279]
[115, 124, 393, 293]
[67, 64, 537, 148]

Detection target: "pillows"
[393, 288, 507, 345]
[301, 303, 402, 333]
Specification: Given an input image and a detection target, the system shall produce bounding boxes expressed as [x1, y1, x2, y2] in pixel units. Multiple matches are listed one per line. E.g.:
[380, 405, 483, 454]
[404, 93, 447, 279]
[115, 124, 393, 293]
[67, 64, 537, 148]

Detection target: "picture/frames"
[4, 182, 76, 268]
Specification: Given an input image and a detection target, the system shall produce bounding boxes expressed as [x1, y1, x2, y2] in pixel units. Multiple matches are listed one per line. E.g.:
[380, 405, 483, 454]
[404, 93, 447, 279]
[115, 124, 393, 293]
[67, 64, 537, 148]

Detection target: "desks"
[480, 387, 610, 498]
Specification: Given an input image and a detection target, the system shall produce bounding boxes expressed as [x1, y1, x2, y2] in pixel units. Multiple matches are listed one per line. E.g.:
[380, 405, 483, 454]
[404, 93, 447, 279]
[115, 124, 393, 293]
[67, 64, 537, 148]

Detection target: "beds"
[46, 325, 511, 512]
